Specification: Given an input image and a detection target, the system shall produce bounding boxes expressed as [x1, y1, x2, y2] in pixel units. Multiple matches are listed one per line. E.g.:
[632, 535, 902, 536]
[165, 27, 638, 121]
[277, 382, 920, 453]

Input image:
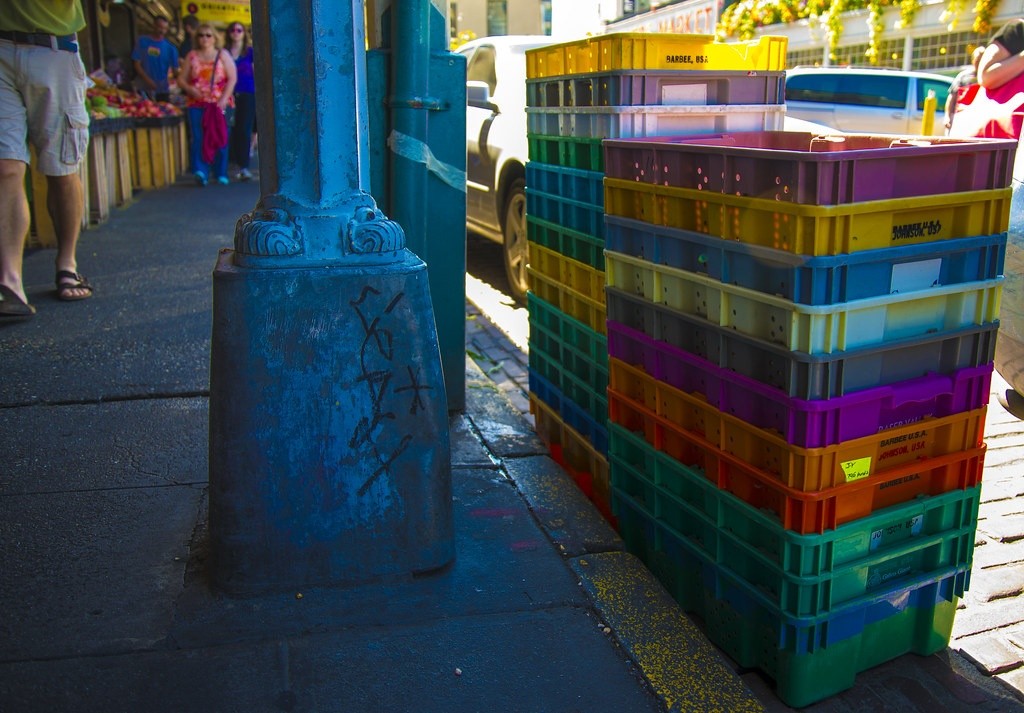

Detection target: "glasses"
[198, 33, 212, 37]
[231, 29, 243, 33]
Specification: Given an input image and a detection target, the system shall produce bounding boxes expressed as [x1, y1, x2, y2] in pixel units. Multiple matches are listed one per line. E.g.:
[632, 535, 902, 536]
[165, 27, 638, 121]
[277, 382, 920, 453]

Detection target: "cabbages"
[86, 95, 119, 118]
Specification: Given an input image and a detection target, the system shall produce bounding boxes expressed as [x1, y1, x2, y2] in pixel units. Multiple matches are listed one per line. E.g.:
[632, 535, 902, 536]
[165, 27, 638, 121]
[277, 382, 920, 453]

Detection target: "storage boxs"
[522, 31, 1019, 710]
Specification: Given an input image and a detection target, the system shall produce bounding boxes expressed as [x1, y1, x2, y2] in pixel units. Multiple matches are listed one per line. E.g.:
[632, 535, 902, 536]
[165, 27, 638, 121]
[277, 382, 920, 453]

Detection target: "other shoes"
[195, 171, 207, 187]
[995, 388, 1024, 419]
[217, 175, 230, 185]
[238, 167, 252, 179]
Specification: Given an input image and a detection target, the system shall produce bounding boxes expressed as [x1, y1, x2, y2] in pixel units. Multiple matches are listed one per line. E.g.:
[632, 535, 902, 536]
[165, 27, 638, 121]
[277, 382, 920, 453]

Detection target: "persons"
[943, 19, 1024, 137]
[0, 0, 255, 317]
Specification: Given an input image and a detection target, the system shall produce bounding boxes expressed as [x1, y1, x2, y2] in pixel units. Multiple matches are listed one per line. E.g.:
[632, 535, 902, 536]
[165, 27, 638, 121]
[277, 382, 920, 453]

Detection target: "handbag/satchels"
[950, 73, 1024, 146]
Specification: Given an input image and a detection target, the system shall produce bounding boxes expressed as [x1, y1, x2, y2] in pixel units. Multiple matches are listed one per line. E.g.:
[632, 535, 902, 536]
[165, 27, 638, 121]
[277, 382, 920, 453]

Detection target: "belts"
[0, 31, 78, 55]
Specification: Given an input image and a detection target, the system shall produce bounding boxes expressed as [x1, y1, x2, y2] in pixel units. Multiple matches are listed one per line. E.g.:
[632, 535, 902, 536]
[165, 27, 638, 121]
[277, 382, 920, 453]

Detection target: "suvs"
[780, 62, 957, 140]
[455, 35, 576, 305]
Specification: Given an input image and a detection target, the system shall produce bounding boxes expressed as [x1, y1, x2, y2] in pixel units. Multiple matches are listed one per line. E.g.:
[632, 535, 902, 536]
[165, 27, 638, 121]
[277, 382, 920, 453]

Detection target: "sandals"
[0, 285, 36, 316]
[56, 270, 93, 300]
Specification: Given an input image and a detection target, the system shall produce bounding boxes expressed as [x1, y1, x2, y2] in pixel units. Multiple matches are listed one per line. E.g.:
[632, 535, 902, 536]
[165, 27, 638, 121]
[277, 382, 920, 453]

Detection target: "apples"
[117, 97, 180, 117]
